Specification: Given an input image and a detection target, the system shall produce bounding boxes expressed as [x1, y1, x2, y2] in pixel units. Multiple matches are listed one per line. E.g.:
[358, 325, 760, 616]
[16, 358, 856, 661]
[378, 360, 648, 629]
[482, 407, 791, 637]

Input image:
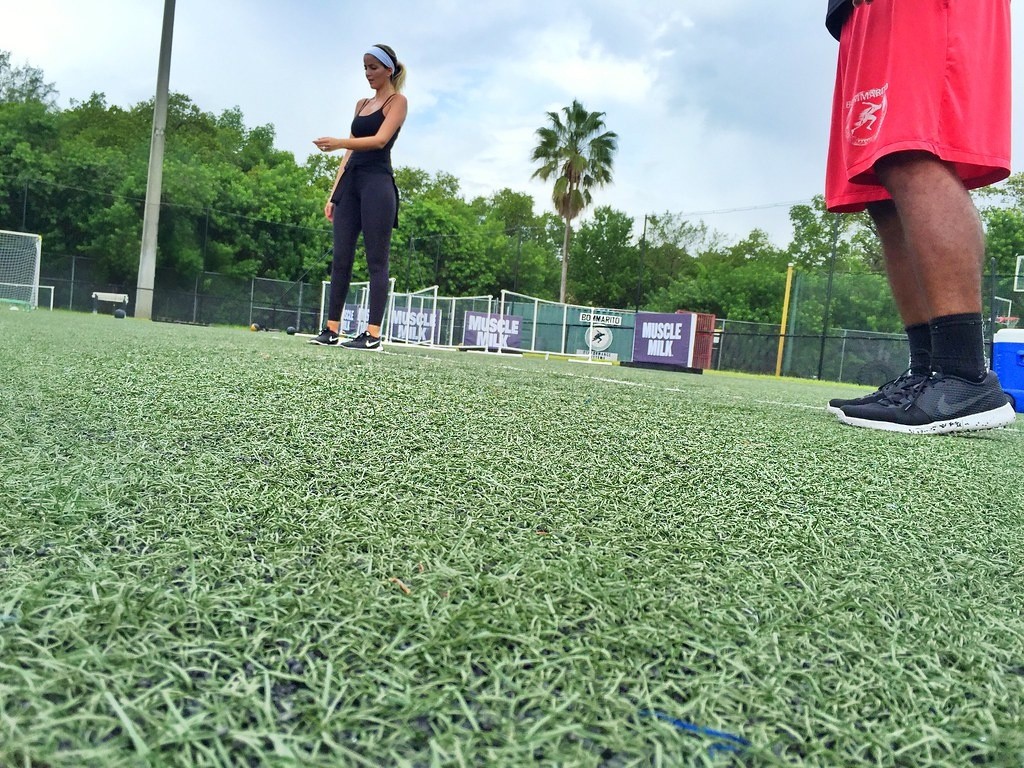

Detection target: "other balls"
[251, 324, 260, 331]
[115, 309, 126, 318]
[286, 327, 296, 336]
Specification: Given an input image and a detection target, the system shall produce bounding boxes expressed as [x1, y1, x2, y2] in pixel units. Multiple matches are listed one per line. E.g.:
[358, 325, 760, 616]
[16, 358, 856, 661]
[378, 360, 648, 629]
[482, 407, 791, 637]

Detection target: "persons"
[307, 44, 407, 352]
[826, 0, 1016, 435]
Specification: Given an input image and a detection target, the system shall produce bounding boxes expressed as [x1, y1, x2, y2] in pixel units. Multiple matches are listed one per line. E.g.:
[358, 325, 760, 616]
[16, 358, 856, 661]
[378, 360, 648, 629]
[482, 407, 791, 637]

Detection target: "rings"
[323, 146, 325, 150]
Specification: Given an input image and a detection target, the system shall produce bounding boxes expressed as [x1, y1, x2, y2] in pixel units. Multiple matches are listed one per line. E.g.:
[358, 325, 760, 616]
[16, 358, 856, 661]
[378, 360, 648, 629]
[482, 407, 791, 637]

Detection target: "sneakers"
[309, 327, 341, 346]
[828, 373, 920, 413]
[342, 332, 384, 351]
[838, 349, 1017, 435]
